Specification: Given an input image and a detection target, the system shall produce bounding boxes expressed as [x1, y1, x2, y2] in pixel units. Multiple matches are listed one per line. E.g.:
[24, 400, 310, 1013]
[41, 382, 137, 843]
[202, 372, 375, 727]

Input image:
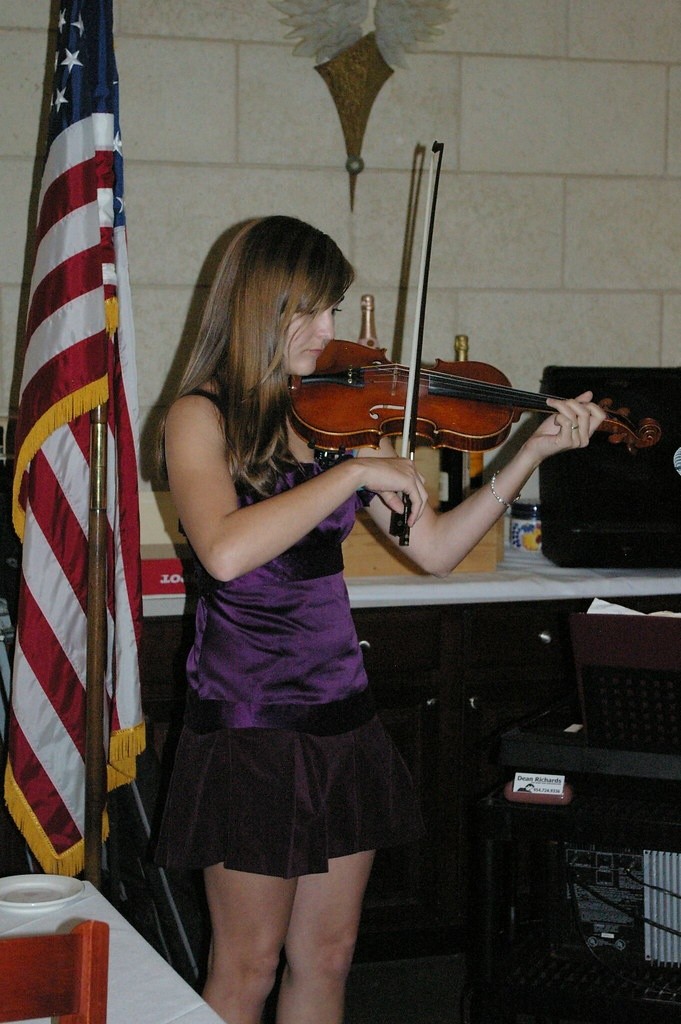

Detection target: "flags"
[4, 1, 144, 878]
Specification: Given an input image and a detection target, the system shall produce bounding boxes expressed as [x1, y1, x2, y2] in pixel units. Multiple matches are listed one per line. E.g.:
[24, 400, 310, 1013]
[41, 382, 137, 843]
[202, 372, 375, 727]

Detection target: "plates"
[0, 874, 85, 913]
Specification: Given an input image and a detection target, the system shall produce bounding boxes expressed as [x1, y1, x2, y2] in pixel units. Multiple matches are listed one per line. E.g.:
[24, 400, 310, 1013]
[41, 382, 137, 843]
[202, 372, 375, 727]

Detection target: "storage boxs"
[340, 512, 506, 578]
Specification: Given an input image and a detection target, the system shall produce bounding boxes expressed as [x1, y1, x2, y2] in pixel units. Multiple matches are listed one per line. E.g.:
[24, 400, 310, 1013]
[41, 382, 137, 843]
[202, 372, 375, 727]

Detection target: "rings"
[572, 425, 579, 430]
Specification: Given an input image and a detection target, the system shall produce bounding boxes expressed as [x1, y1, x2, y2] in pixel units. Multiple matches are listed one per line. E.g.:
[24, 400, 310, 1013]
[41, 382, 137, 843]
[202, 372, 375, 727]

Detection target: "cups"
[508, 499, 544, 554]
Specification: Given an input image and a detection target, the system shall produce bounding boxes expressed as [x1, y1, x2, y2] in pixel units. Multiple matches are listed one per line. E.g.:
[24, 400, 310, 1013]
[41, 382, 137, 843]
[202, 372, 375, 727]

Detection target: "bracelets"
[490, 471, 521, 509]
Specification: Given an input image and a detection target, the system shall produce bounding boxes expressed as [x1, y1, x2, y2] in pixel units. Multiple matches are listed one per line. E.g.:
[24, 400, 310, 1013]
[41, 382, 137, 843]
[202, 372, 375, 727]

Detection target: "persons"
[140, 216, 606, 1024]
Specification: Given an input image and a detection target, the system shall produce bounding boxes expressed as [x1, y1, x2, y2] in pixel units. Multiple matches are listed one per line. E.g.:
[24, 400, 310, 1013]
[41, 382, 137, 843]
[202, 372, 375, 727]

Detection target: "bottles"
[439, 335, 483, 513]
[357, 294, 380, 350]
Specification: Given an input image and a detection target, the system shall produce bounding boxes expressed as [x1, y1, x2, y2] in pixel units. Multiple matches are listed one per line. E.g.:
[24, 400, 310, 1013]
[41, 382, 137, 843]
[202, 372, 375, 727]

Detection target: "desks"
[0, 880, 225, 1024]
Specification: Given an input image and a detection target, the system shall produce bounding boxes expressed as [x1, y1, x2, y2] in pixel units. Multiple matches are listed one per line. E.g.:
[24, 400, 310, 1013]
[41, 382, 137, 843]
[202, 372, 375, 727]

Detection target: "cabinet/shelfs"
[130, 542, 680, 963]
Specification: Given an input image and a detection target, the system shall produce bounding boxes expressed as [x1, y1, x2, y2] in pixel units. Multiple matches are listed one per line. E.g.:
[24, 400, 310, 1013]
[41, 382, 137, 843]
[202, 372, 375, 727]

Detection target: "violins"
[289, 338, 663, 457]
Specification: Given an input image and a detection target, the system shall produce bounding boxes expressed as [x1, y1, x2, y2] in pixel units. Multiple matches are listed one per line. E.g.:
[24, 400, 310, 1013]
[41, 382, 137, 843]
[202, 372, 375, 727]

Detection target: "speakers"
[540, 366, 681, 569]
